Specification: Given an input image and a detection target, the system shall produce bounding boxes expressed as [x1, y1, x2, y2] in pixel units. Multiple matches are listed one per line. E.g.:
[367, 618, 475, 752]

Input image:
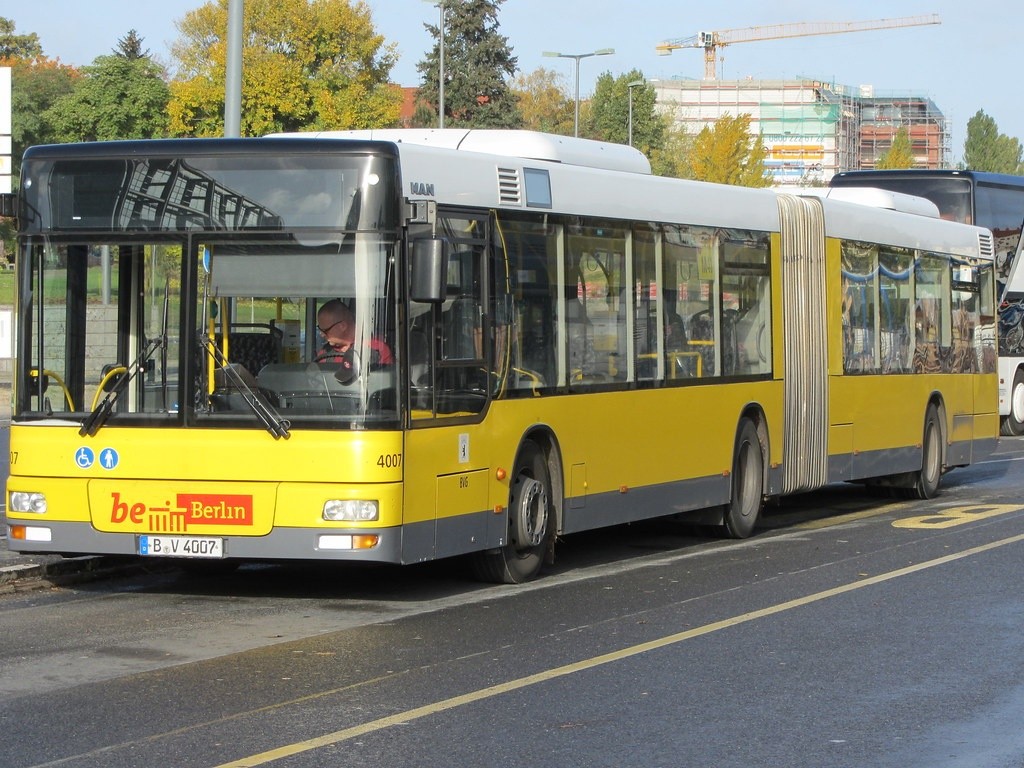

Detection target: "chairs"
[411, 330, 432, 409]
[690, 310, 736, 373]
[196, 323, 284, 387]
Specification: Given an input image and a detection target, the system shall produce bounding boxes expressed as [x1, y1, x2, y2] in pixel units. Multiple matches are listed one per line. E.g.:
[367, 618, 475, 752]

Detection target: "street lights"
[540, 49, 617, 138]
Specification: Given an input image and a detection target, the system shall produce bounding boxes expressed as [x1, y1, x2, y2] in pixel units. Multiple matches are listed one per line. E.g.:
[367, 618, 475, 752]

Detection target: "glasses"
[316, 319, 345, 334]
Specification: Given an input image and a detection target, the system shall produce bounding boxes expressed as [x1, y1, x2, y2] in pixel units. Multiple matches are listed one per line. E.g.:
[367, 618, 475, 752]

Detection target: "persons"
[310, 299, 394, 365]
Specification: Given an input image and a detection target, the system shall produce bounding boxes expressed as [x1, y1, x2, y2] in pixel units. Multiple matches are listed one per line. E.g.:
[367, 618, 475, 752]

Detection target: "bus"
[7, 130, 1002, 584]
[829, 168, 1024, 435]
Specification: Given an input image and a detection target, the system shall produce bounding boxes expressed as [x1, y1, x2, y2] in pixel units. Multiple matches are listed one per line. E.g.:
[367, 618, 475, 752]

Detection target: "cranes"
[652, 13, 944, 84]
